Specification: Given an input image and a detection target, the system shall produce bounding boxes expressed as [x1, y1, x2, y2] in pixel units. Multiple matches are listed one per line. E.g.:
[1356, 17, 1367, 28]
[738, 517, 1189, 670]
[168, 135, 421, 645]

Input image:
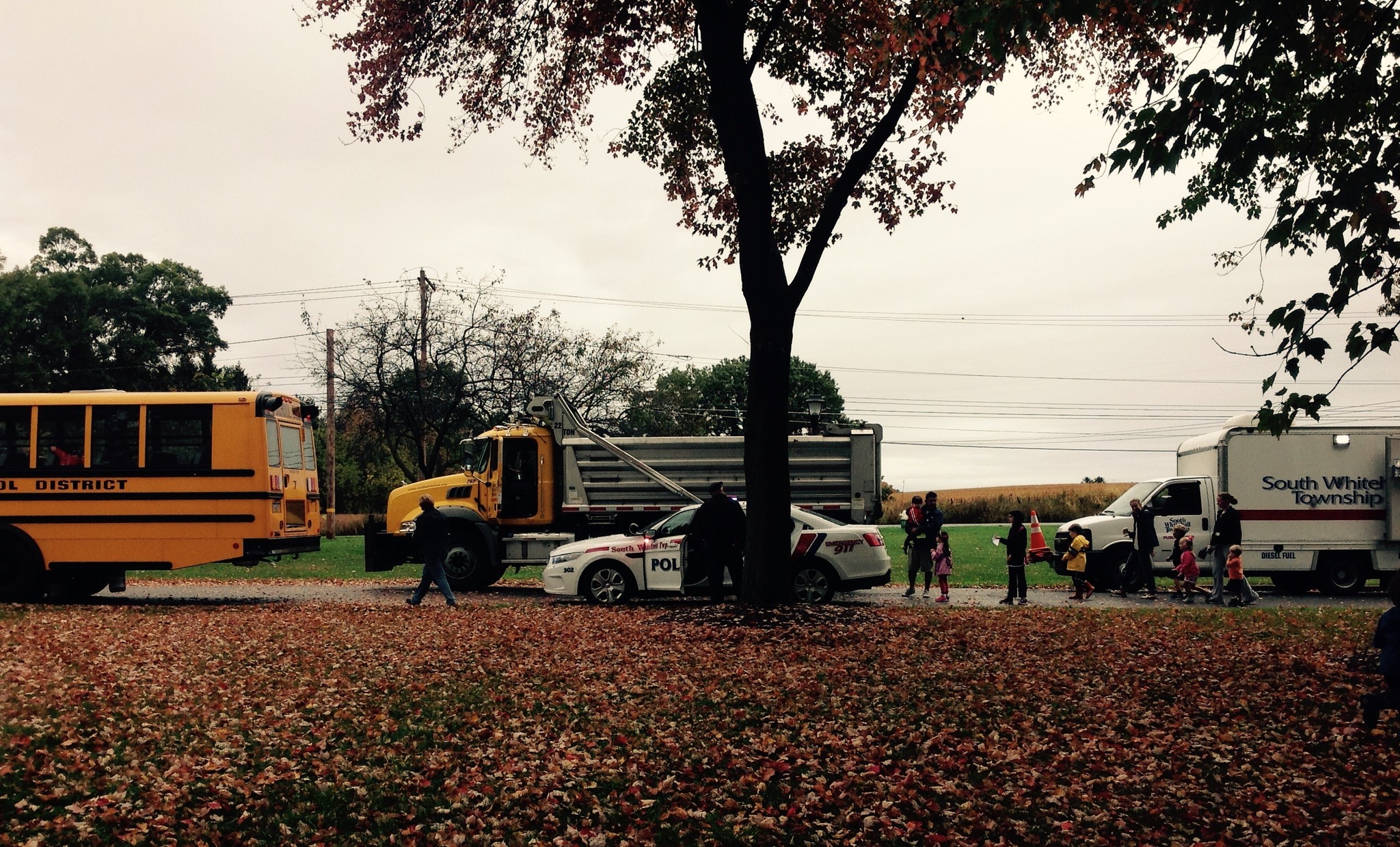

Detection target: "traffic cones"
[1026, 511, 1055, 564]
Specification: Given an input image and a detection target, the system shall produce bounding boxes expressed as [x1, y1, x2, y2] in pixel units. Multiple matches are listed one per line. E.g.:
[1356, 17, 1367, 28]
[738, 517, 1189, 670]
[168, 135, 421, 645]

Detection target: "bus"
[0, 388, 323, 604]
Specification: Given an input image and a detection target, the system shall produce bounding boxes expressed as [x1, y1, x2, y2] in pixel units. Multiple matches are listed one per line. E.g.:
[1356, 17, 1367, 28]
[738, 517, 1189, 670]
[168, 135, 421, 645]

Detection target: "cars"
[542, 500, 891, 607]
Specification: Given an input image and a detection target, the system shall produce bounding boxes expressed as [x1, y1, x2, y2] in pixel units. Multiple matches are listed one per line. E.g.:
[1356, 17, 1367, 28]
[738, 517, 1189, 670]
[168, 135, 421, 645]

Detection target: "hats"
[709, 482, 723, 493]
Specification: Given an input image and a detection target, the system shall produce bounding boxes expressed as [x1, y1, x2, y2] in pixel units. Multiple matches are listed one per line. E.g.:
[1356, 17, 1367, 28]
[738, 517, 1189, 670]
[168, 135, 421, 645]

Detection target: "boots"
[1082, 580, 1095, 599]
[1068, 586, 1083, 599]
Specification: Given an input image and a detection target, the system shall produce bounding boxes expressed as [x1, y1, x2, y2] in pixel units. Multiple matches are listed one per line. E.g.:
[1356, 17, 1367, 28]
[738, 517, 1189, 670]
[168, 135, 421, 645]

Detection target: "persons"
[1111, 499, 1161, 600]
[899, 491, 945, 598]
[995, 510, 1028, 605]
[1062, 524, 1095, 600]
[406, 494, 458, 608]
[1166, 524, 1212, 604]
[687, 481, 747, 606]
[930, 530, 953, 602]
[1206, 492, 1243, 604]
[1227, 544, 1245, 606]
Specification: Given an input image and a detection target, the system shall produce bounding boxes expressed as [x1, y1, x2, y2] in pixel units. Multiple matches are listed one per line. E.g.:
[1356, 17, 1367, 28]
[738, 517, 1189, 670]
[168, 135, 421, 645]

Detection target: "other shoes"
[910, 541, 915, 548]
[922, 589, 930, 598]
[901, 589, 916, 597]
[406, 598, 412, 604]
[1209, 598, 1224, 604]
[1205, 591, 1213, 601]
[1018, 597, 1027, 605]
[1111, 589, 1127, 598]
[999, 597, 1014, 604]
[1141, 593, 1157, 599]
[901, 545, 907, 555]
[1170, 594, 1184, 599]
[1360, 693, 1380, 728]
[1182, 597, 1195, 603]
[936, 595, 950, 602]
[1228, 598, 1236, 606]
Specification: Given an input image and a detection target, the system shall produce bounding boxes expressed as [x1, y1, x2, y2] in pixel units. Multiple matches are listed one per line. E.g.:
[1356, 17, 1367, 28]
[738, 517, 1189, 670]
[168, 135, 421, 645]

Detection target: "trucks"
[364, 394, 885, 592]
[1055, 413, 1400, 597]
